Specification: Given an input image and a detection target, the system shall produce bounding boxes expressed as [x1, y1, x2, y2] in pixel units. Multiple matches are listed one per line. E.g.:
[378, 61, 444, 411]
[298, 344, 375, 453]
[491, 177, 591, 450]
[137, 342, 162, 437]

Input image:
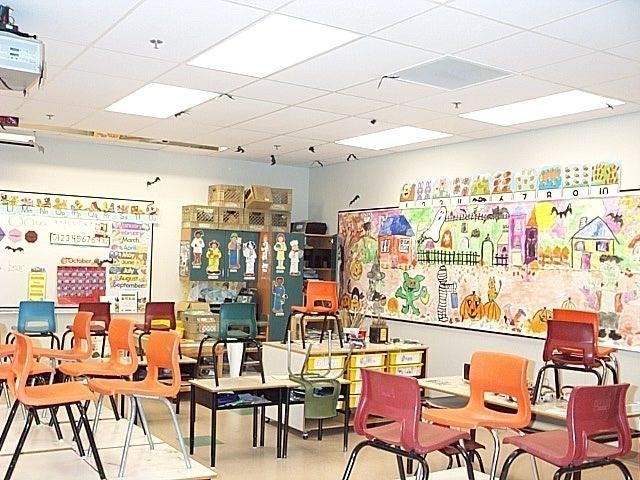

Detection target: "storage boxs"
[182, 184, 293, 233]
[182, 309, 221, 342]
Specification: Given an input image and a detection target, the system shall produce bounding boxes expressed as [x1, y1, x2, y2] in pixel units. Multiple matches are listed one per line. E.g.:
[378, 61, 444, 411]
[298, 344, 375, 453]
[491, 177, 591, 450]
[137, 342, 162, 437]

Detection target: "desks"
[1, 400, 217, 480]
[133, 332, 261, 378]
[260, 374, 353, 458]
[190, 374, 287, 468]
[261, 336, 430, 440]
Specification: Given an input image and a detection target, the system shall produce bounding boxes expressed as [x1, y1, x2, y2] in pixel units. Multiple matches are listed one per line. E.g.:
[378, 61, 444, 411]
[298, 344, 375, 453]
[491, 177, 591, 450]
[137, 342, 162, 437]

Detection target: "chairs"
[1, 301, 191, 480]
[341, 349, 634, 480]
[199, 302, 265, 387]
[531, 309, 619, 405]
[287, 330, 354, 418]
[281, 280, 343, 350]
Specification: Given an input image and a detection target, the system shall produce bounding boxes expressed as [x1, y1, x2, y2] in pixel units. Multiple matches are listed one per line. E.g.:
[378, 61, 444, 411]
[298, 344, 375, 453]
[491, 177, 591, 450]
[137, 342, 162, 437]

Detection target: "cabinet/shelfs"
[180, 229, 339, 342]
